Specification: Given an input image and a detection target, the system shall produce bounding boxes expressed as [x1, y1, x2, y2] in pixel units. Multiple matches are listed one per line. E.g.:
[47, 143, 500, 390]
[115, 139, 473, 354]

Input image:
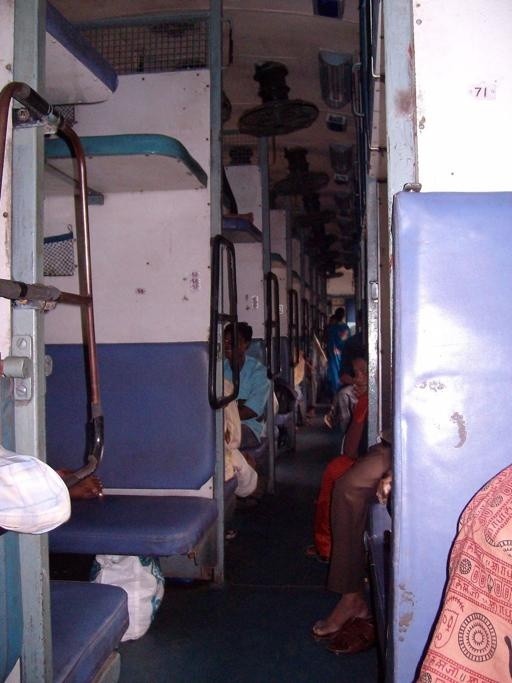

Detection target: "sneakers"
[309, 614, 339, 642]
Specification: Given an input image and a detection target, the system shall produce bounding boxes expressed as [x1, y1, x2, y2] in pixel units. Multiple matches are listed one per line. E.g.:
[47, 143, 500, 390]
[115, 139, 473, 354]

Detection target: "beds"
[221, 213, 325, 313]
[43, 0, 205, 189]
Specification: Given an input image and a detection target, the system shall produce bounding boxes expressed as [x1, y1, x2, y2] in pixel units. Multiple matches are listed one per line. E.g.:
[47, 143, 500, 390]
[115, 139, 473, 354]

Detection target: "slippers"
[326, 615, 376, 657]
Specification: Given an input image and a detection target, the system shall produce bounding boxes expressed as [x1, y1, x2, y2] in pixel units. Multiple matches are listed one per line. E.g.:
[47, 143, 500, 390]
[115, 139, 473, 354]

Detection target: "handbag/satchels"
[91, 554, 169, 643]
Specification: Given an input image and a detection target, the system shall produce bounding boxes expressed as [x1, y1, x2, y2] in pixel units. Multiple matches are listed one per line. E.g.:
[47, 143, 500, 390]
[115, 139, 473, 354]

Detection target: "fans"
[239, 61, 345, 277]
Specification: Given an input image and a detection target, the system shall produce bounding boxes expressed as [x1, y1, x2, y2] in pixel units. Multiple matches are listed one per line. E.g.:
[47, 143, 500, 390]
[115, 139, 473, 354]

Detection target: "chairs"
[43, 342, 219, 584]
[392, 191, 511, 682]
[247, 341, 271, 457]
[47, 578, 132, 680]
[365, 497, 391, 647]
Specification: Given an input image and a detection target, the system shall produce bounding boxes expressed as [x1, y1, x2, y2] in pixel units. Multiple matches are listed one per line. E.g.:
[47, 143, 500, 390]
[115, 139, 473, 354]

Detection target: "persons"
[222, 308, 391, 637]
[56, 469, 103, 500]
